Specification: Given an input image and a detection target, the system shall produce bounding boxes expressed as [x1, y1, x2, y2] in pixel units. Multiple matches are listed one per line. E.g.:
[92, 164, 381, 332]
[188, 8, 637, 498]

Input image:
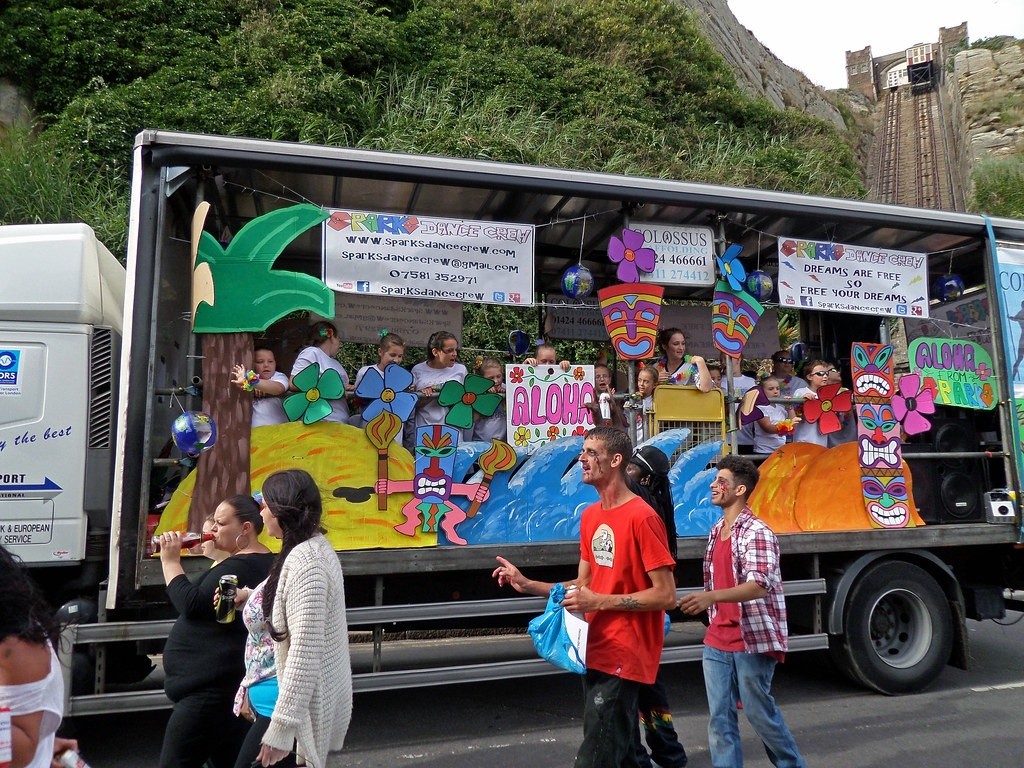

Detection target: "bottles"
[151, 531, 216, 553]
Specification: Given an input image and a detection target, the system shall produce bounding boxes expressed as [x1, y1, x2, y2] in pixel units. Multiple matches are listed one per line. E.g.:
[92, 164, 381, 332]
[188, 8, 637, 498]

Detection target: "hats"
[630, 446, 670, 478]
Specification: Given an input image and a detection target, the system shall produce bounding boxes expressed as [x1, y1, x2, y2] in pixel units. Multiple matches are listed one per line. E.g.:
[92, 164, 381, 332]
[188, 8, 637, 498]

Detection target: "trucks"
[1, 133, 1024, 721]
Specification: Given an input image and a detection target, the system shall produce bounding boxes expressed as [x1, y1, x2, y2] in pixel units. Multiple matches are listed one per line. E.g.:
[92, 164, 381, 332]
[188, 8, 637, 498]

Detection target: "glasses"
[632, 448, 653, 471]
[828, 368, 838, 373]
[810, 371, 829, 378]
[774, 357, 793, 364]
[594, 374, 611, 381]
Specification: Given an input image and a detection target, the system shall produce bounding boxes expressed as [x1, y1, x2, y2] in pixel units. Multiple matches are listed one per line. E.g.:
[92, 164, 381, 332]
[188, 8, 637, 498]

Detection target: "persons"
[213, 469, 353, 768]
[522, 342, 570, 372]
[623, 367, 659, 444]
[231, 345, 289, 427]
[624, 446, 688, 768]
[201, 512, 231, 569]
[492, 424, 677, 768]
[289, 321, 355, 423]
[158, 492, 275, 768]
[719, 348, 857, 456]
[0, 543, 80, 768]
[353, 332, 405, 389]
[677, 455, 809, 768]
[594, 363, 615, 395]
[411, 330, 468, 442]
[472, 355, 507, 441]
[653, 328, 712, 392]
[707, 364, 723, 388]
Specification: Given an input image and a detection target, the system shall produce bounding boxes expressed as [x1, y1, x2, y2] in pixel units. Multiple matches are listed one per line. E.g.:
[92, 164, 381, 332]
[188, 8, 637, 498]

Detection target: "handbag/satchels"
[527, 582, 587, 674]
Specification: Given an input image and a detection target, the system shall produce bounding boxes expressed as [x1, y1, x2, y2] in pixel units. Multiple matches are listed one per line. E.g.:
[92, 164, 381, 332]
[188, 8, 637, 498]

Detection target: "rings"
[166, 539, 170, 542]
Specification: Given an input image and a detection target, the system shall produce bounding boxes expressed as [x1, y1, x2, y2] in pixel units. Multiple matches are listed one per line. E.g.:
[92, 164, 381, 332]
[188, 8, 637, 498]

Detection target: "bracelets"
[776, 419, 795, 435]
[241, 368, 260, 391]
[785, 405, 792, 411]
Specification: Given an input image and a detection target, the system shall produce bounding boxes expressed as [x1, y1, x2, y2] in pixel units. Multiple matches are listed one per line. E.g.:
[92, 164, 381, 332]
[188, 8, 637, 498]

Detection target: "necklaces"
[770, 372, 793, 388]
[656, 355, 698, 383]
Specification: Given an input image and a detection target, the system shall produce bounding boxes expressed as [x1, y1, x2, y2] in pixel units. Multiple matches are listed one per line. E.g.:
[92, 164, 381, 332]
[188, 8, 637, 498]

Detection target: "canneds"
[55, 749, 91, 768]
[216, 575, 238, 624]
